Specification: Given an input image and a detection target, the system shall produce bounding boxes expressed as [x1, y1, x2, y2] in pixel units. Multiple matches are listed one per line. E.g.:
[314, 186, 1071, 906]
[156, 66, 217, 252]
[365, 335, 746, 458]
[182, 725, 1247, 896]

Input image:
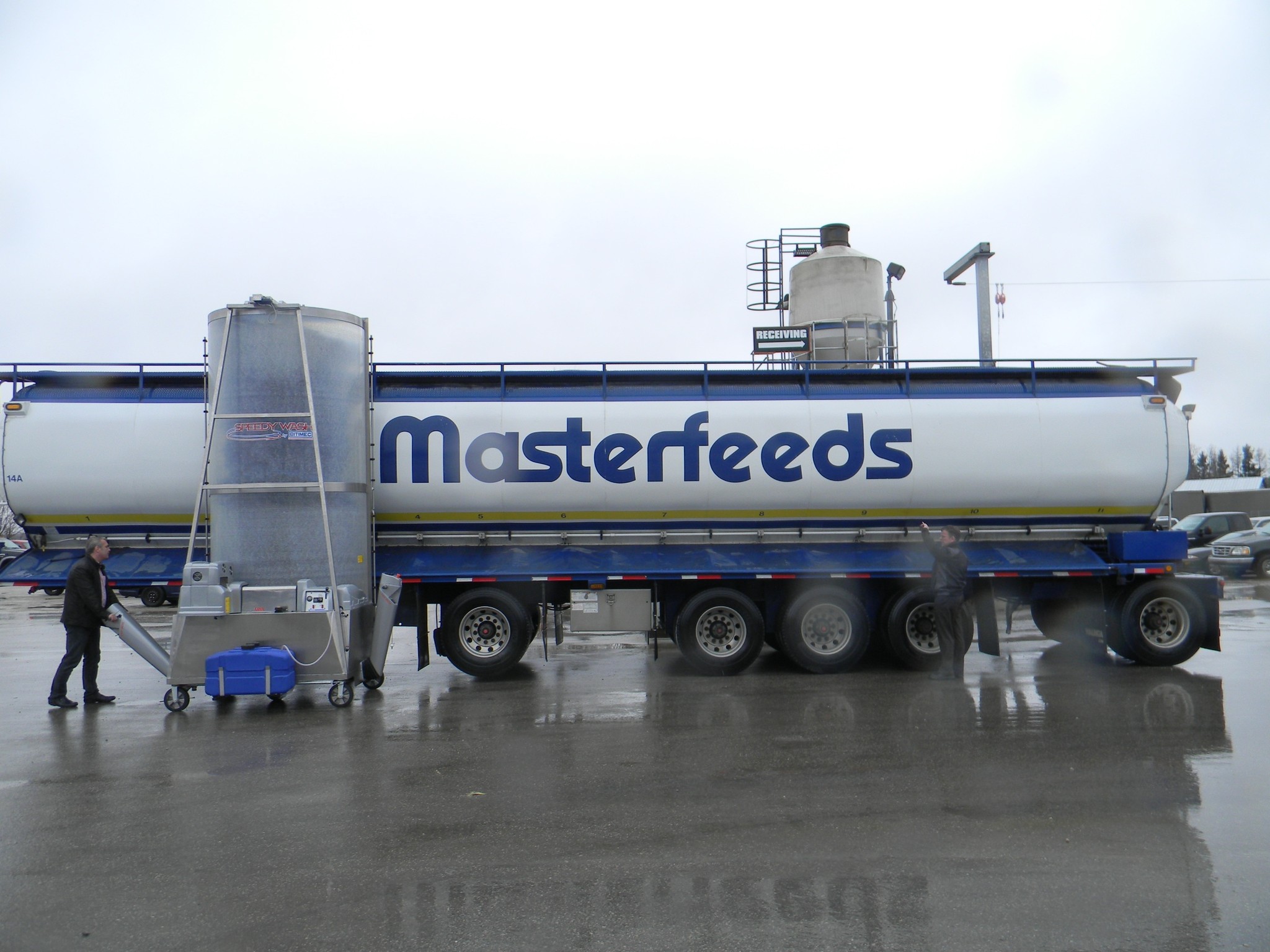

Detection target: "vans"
[1169, 511, 1253, 549]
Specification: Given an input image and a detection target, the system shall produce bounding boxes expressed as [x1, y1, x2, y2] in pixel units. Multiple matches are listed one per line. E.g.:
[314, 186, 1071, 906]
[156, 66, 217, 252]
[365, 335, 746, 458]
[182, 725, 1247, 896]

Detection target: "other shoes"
[929, 672, 963, 680]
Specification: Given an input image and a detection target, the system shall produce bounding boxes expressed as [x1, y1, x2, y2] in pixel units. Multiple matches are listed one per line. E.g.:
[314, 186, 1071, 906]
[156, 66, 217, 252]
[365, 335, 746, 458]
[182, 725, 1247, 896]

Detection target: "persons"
[47, 536, 128, 708]
[921, 522, 971, 682]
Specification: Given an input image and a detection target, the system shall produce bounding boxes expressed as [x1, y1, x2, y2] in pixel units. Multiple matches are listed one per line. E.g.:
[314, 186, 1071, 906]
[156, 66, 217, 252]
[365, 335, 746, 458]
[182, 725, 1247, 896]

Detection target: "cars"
[1153, 515, 1270, 580]
[0, 537, 31, 571]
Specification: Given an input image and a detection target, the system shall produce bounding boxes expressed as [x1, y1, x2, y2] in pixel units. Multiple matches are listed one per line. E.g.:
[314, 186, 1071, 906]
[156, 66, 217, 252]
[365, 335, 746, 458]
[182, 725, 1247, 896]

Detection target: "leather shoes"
[48, 697, 78, 706]
[84, 692, 115, 702]
